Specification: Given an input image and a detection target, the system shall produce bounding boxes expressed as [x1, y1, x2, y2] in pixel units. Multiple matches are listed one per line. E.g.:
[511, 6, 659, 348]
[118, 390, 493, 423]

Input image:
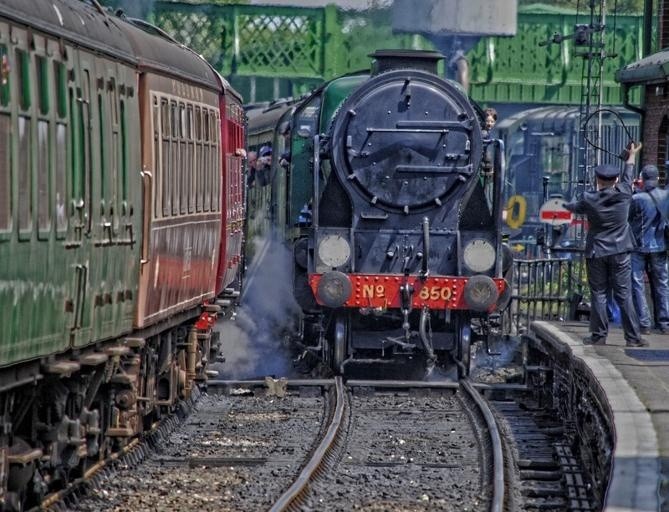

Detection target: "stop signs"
[539, 197, 574, 226]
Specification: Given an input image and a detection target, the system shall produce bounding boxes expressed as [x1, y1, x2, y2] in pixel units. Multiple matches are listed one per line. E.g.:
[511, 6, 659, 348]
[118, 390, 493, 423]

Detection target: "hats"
[595, 165, 620, 181]
[642, 164, 659, 188]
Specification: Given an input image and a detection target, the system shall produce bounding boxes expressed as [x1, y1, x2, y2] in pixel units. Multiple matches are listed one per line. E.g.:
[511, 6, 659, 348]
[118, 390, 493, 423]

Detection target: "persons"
[484, 108, 498, 131]
[254, 145, 272, 186]
[562, 142, 650, 348]
[278, 150, 291, 169]
[629, 159, 668, 335]
[247, 150, 259, 184]
[632, 169, 645, 193]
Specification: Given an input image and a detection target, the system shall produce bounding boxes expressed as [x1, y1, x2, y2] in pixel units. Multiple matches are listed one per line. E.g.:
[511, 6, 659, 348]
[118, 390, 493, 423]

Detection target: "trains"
[243, 45, 518, 387]
[0, 0, 251, 512]
[498, 100, 641, 287]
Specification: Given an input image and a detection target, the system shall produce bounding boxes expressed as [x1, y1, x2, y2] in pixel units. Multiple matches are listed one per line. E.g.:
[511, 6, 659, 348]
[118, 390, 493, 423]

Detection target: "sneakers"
[582, 336, 606, 346]
[627, 338, 650, 348]
[641, 326, 669, 336]
